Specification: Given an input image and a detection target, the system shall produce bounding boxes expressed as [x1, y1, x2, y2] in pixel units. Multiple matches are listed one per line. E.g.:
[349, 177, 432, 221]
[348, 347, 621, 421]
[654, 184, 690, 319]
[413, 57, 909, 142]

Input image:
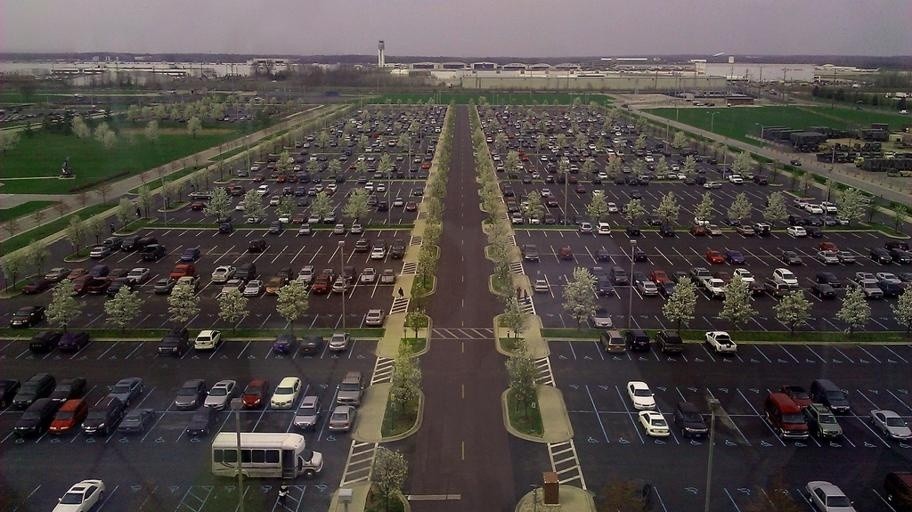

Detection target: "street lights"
[337, 240, 347, 332]
[703, 396, 720, 508]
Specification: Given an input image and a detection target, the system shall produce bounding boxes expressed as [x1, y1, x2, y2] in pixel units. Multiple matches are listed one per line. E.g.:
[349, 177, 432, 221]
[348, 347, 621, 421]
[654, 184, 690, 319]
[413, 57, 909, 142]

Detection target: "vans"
[898, 109, 909, 116]
[211, 431, 324, 485]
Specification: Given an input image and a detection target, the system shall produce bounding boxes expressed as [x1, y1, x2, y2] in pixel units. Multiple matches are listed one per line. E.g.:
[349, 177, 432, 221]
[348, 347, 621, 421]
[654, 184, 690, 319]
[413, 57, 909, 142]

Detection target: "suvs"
[155, 333, 189, 358]
[30, 329, 63, 353]
[194, 329, 222, 351]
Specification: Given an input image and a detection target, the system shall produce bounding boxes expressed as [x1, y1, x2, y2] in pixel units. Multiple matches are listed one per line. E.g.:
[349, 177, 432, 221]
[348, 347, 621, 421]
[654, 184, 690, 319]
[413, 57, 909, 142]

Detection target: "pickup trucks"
[10, 305, 46, 328]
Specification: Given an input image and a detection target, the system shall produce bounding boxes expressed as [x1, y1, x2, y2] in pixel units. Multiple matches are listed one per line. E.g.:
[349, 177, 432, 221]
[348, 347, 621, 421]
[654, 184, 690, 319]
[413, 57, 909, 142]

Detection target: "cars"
[885, 469, 911, 510]
[299, 334, 325, 354]
[365, 308, 385, 325]
[328, 332, 352, 352]
[58, 330, 90, 353]
[51, 478, 106, 511]
[272, 333, 297, 355]
[884, 92, 912, 99]
[5, 102, 110, 123]
[192, 105, 449, 235]
[626, 378, 911, 447]
[759, 86, 777, 96]
[805, 479, 857, 511]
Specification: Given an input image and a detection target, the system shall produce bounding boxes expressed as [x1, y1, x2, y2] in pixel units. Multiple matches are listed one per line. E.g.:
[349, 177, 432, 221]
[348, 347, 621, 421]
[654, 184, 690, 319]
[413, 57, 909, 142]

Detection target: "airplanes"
[850, 66, 881, 73]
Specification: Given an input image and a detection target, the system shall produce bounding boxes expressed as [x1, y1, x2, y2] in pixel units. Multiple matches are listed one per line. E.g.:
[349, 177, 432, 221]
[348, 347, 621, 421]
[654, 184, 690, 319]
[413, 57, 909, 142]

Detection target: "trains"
[48, 67, 186, 79]
